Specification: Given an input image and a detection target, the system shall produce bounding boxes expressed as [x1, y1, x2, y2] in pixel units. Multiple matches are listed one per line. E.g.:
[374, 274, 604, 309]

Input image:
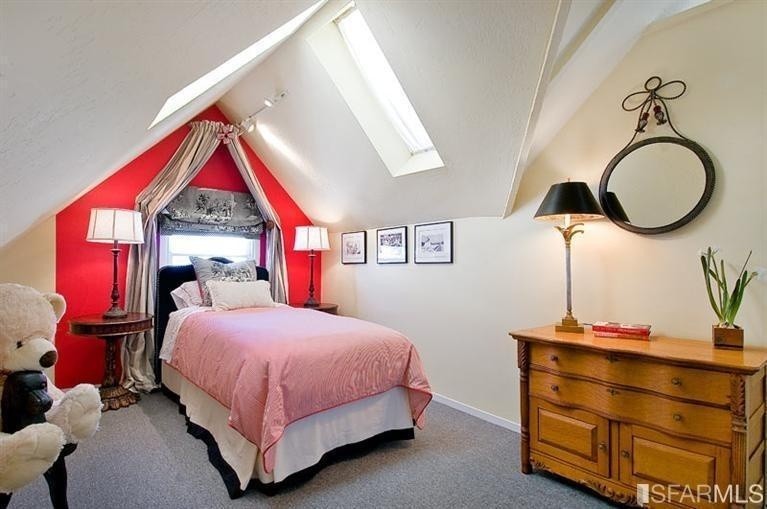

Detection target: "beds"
[68, 312, 153, 412]
[154, 257, 432, 500]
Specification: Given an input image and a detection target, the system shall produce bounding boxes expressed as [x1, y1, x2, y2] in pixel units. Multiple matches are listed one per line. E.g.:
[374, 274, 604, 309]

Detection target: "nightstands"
[290, 302, 338, 316]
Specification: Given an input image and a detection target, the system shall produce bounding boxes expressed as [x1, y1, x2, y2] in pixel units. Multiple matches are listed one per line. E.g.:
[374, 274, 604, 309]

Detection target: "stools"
[1, 442, 79, 509]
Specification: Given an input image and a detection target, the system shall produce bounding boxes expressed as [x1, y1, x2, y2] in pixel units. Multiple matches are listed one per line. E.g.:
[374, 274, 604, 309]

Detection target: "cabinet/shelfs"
[508, 321, 766, 509]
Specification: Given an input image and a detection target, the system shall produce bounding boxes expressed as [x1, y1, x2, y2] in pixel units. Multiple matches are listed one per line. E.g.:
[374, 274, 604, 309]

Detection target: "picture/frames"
[340, 230, 366, 264]
[414, 221, 453, 264]
[376, 226, 407, 264]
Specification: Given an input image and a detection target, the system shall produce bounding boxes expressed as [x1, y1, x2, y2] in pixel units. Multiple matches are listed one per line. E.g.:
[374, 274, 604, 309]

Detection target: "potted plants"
[700, 246, 758, 349]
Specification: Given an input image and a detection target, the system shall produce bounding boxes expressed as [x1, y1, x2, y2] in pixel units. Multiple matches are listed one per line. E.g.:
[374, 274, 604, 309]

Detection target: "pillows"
[189, 255, 257, 306]
[206, 280, 275, 311]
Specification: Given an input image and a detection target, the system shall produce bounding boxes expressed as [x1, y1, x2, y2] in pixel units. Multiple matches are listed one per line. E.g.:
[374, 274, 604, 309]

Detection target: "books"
[593, 320, 651, 340]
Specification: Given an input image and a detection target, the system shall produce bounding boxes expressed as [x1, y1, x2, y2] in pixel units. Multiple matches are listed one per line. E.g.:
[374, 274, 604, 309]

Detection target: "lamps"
[237, 97, 275, 137]
[292, 226, 330, 307]
[606, 191, 629, 224]
[533, 178, 604, 334]
[85, 208, 144, 321]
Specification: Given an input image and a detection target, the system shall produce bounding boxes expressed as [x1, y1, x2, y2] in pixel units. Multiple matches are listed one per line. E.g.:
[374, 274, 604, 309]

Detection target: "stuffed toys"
[0, 282, 101, 497]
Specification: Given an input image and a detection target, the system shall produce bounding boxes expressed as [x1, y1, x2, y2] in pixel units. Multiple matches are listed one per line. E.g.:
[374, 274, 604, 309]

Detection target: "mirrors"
[598, 77, 716, 236]
